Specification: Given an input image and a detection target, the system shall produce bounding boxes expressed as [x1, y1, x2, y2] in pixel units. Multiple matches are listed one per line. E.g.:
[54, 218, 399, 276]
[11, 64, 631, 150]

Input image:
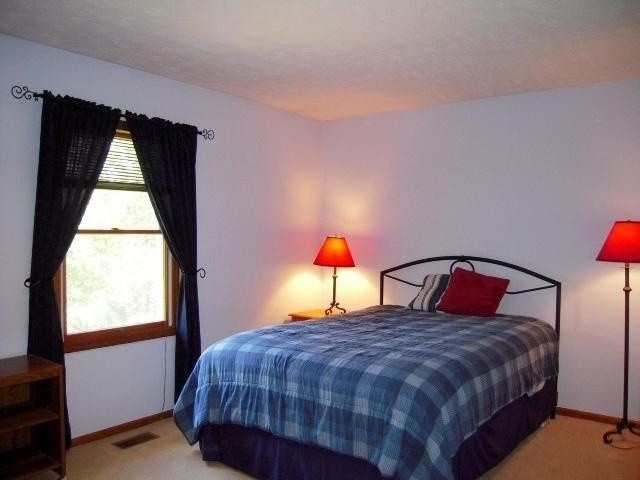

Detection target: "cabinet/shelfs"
[0, 354, 66, 480]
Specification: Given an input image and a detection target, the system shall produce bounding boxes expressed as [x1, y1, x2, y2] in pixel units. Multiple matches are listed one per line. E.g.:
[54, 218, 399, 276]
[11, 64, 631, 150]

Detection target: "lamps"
[313, 236, 356, 315]
[596, 221, 639, 444]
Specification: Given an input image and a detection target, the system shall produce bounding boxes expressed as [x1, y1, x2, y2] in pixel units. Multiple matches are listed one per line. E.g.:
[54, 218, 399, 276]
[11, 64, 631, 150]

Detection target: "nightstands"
[288, 308, 346, 322]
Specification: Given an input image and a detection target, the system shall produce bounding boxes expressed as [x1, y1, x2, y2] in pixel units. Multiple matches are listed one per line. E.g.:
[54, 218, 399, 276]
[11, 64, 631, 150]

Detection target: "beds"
[193, 255, 561, 480]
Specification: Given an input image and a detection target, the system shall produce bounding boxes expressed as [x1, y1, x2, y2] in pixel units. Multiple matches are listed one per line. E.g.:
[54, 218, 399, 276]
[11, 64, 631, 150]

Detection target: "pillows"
[408, 268, 510, 317]
